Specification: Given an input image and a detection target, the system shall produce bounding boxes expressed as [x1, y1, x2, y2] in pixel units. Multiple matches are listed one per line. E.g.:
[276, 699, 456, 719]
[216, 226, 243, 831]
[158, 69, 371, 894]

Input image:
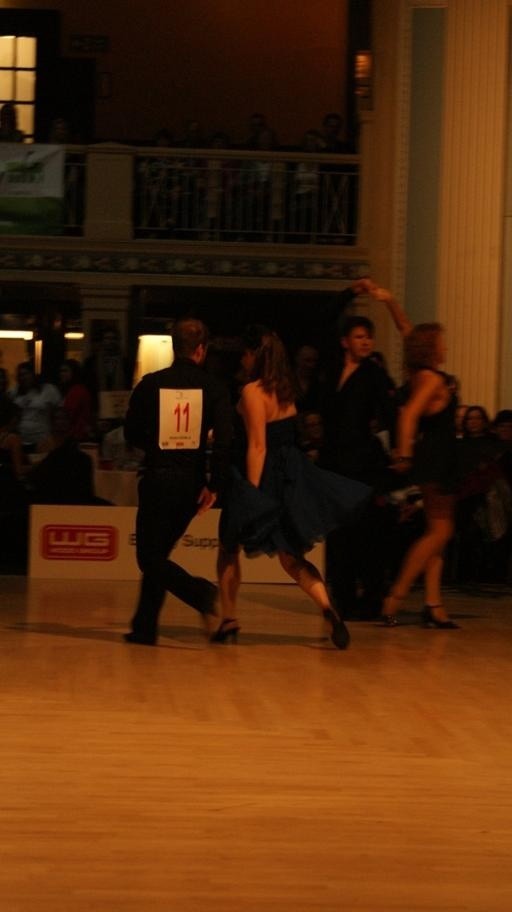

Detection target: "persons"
[311, 278, 400, 621]
[366, 281, 464, 630]
[451, 401, 509, 593]
[289, 348, 327, 466]
[210, 332, 350, 648]
[82, 326, 137, 441]
[1, 317, 95, 575]
[364, 351, 425, 525]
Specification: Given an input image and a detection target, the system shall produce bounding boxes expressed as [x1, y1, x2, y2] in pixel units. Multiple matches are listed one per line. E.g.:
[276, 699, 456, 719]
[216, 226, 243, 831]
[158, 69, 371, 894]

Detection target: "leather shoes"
[203, 589, 222, 634]
[124, 629, 158, 645]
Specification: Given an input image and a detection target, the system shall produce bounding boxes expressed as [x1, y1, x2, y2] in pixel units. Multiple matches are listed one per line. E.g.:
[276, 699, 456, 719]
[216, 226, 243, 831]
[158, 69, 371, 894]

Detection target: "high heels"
[325, 607, 350, 649]
[210, 618, 242, 643]
[422, 604, 461, 630]
[379, 593, 398, 626]
